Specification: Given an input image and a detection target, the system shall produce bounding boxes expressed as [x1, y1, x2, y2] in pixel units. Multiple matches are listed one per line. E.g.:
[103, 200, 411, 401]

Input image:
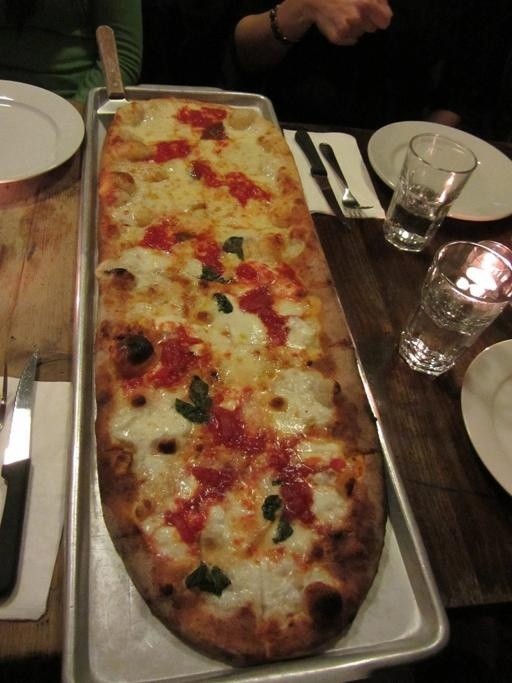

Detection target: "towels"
[0, 376, 74, 620]
[283, 129, 387, 220]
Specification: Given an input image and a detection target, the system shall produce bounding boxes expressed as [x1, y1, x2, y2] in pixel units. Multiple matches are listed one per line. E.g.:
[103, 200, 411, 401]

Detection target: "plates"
[461, 338, 512, 498]
[0, 78, 85, 185]
[368, 119, 512, 221]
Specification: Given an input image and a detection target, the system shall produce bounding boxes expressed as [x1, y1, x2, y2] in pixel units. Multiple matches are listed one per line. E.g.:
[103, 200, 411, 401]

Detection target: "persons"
[0, 1, 144, 103]
[161, 0, 486, 137]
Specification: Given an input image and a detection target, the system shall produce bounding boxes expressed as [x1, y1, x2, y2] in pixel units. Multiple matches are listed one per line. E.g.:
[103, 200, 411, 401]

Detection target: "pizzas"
[95, 96, 386, 664]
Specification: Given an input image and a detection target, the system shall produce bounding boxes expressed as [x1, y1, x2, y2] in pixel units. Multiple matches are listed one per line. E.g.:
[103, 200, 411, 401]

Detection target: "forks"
[319, 142, 365, 219]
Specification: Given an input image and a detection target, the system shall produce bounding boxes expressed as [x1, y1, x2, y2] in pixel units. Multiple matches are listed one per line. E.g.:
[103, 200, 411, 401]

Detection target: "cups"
[381, 134, 512, 378]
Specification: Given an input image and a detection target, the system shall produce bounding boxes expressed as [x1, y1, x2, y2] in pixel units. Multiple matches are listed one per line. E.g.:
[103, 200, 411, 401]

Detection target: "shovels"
[96, 25, 131, 183]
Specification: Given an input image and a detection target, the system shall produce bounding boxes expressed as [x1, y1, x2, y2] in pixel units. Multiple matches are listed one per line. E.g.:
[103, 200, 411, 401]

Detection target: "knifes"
[297, 129, 351, 230]
[0, 349, 43, 600]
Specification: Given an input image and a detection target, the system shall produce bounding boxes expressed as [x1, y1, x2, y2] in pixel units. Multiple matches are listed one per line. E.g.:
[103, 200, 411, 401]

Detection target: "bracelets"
[270, 5, 298, 46]
